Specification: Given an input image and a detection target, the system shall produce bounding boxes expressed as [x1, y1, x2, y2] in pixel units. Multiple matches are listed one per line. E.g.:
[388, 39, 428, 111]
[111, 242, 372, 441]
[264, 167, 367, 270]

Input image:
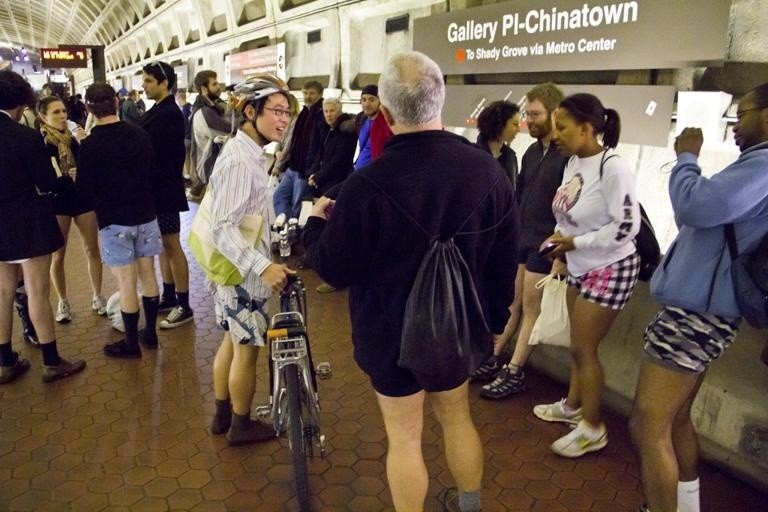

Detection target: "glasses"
[149, 60, 170, 84]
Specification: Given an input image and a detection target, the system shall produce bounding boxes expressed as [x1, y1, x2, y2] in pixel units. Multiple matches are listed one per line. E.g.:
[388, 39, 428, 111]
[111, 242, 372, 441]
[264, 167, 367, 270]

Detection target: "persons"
[272, 80, 395, 295]
[190, 69, 297, 444]
[0, 61, 191, 384]
[301, 50, 521, 512]
[469, 82, 644, 457]
[627, 80, 767, 510]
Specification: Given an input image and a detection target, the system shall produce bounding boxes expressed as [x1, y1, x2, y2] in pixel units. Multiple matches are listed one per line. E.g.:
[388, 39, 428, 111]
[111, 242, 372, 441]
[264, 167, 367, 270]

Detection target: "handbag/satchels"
[527, 274, 573, 347]
[188, 206, 262, 287]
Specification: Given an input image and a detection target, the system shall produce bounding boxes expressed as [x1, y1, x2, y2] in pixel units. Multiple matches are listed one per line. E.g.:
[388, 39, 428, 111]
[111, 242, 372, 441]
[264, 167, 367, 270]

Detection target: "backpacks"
[724, 224, 767, 328]
[359, 164, 507, 375]
[603, 154, 661, 281]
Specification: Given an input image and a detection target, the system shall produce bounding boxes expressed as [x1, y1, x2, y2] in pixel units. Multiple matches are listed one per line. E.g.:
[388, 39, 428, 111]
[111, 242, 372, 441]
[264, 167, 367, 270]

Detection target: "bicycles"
[258, 217, 333, 512]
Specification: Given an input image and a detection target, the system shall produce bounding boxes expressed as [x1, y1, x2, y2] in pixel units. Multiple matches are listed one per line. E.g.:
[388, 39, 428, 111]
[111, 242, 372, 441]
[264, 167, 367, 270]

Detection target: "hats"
[362, 84, 378, 98]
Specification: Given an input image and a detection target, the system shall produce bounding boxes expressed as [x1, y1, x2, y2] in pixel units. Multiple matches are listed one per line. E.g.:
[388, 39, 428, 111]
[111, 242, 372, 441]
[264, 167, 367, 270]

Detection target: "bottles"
[277, 231, 290, 257]
[272, 211, 285, 230]
[65, 118, 87, 145]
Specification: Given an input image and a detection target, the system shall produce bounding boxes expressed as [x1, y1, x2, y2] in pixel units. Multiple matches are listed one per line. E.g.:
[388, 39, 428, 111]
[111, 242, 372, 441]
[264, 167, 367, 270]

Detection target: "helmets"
[234, 74, 289, 98]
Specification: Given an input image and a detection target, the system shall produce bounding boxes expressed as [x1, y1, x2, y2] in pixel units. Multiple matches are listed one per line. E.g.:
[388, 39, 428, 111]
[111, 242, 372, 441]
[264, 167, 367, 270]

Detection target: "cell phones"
[538, 243, 557, 255]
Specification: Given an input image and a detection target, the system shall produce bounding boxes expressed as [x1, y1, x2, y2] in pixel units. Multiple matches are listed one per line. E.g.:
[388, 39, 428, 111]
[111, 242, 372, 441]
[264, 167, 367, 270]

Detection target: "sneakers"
[227, 420, 275, 446]
[443, 487, 482, 511]
[0, 295, 193, 384]
[551, 424, 607, 458]
[471, 360, 497, 381]
[532, 399, 582, 423]
[209, 417, 232, 433]
[481, 368, 527, 397]
[317, 282, 334, 292]
